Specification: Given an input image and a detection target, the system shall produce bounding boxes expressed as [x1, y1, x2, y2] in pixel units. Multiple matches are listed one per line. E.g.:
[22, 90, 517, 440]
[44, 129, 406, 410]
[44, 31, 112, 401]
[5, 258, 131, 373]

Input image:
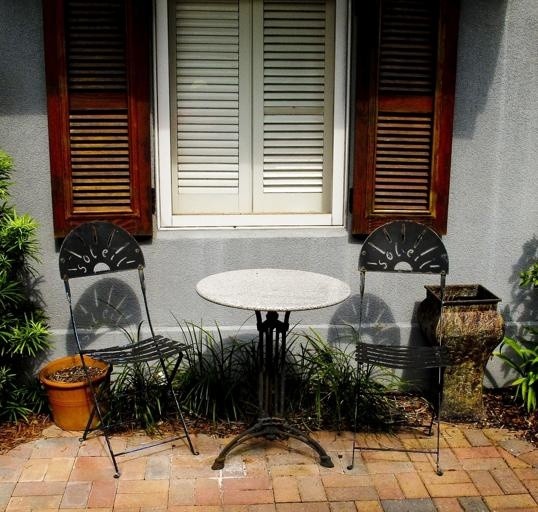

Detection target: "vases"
[417, 283, 506, 423]
[37, 355, 112, 432]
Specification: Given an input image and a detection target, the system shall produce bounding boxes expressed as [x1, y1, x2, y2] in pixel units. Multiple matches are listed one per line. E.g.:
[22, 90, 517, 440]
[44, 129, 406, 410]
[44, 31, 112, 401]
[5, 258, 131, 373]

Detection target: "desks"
[194, 268, 352, 469]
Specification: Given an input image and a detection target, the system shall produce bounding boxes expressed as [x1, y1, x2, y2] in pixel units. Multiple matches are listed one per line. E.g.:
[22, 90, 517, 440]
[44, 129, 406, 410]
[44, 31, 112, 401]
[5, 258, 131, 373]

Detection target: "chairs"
[58, 221, 200, 477]
[346, 220, 449, 476]
